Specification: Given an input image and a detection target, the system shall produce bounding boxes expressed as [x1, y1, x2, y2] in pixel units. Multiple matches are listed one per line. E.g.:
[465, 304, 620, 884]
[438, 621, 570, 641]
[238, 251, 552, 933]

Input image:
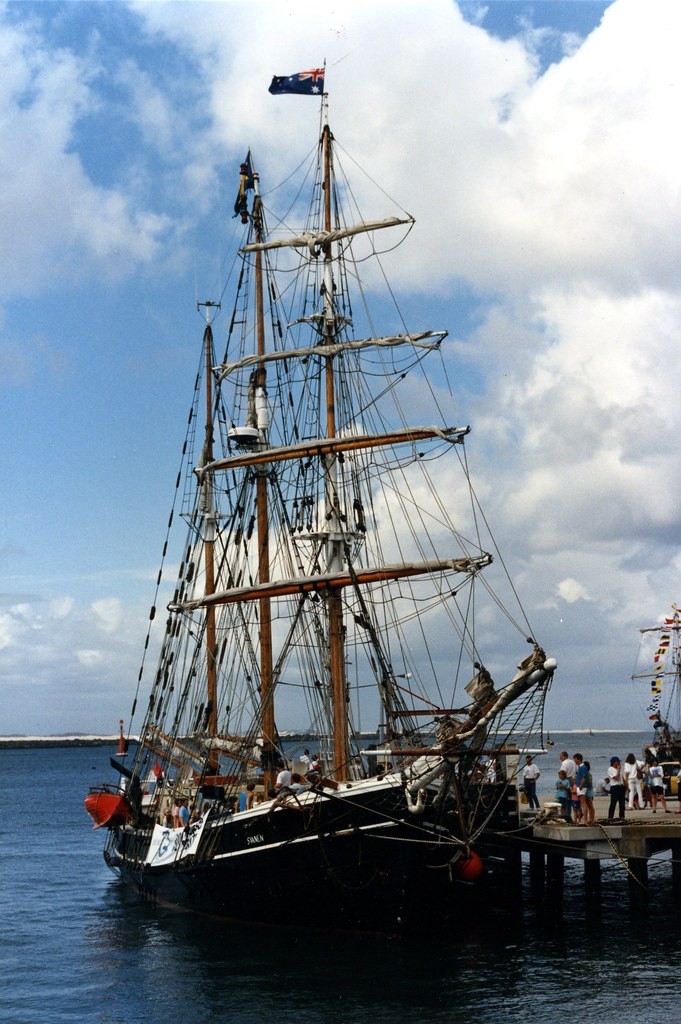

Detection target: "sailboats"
[116, 720, 128, 757]
[84, 58, 557, 930]
[630, 603, 681, 796]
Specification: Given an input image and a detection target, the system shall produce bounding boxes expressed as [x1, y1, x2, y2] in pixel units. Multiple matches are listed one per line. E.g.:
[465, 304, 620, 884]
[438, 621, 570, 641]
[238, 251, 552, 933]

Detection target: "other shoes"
[674, 811, 681, 814]
[665, 810, 671, 813]
[653, 810, 656, 813]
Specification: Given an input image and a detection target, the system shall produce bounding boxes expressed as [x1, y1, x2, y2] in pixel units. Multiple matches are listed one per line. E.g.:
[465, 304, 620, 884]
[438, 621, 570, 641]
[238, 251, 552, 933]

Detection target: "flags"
[233, 152, 256, 219]
[268, 68, 324, 96]
[647, 619, 674, 712]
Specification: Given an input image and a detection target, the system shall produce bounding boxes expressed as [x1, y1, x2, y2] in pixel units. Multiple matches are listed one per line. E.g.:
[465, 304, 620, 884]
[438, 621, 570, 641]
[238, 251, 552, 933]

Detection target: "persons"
[523, 755, 541, 811]
[555, 748, 681, 826]
[161, 750, 321, 827]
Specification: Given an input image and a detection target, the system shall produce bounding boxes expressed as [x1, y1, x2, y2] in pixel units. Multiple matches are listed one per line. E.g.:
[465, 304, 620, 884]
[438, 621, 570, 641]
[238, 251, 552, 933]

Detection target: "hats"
[610, 756, 621, 764]
